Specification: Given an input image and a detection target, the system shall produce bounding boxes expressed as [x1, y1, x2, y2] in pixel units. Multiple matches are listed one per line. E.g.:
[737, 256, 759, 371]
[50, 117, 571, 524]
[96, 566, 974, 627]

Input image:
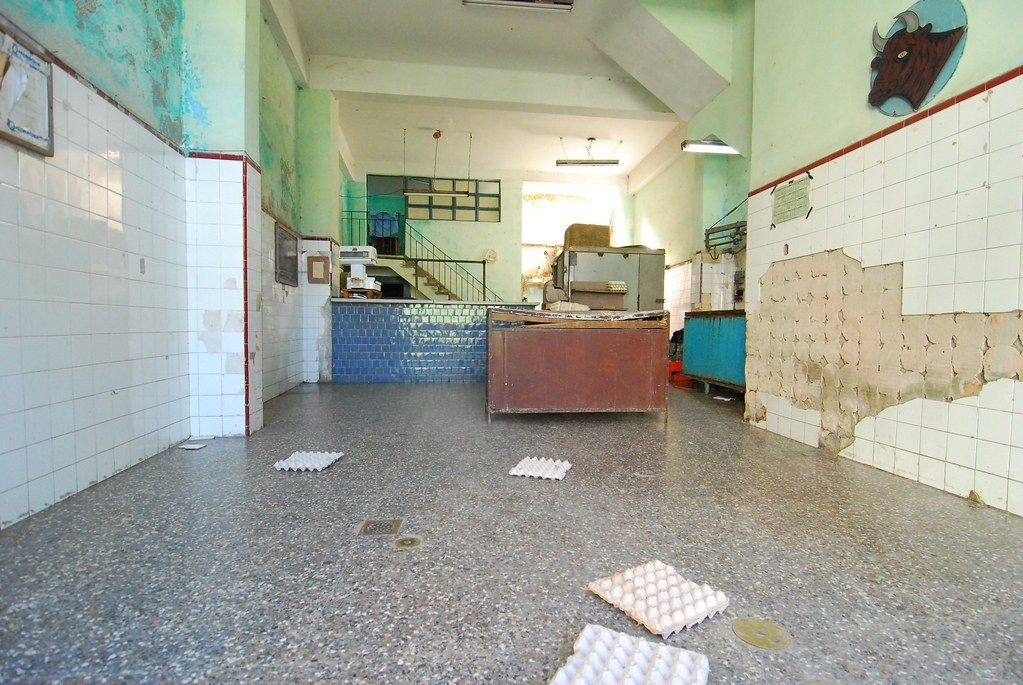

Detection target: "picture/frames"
[0, 24, 57, 158]
[275, 222, 299, 288]
[307, 256, 330, 284]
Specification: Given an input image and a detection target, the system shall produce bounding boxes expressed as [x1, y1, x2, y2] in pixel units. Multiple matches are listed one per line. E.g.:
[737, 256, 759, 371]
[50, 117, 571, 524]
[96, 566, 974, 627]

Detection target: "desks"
[341, 288, 382, 299]
[484, 308, 671, 426]
[681, 309, 746, 393]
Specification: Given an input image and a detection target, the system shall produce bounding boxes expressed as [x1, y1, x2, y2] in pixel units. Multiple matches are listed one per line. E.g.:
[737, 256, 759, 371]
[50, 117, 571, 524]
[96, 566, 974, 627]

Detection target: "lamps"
[680, 139, 740, 155]
[403, 128, 472, 198]
[462, 0, 575, 15]
[556, 137, 623, 167]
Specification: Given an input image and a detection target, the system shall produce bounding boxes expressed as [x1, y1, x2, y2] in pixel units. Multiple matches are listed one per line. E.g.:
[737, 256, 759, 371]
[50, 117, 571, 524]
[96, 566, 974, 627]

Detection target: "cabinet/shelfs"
[554, 246, 665, 311]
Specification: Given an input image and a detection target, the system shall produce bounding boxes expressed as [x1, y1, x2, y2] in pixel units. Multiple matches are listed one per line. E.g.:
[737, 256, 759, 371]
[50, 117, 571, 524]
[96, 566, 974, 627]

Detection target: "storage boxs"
[562, 224, 610, 249]
[570, 281, 628, 312]
